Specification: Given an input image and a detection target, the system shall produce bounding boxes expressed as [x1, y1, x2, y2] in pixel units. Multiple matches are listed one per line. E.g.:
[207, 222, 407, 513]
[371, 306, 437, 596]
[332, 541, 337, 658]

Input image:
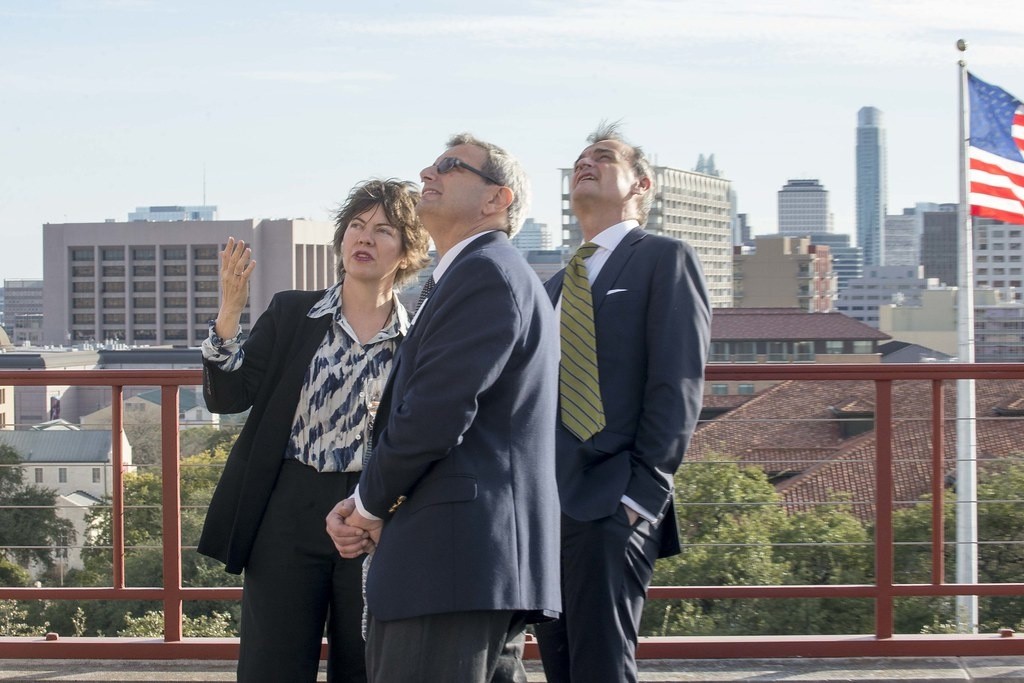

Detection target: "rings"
[233, 272, 242, 277]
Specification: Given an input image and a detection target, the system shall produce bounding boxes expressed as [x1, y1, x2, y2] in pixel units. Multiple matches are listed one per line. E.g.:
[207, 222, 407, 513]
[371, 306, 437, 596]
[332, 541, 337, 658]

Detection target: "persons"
[200, 178, 434, 683]
[326, 131, 562, 683]
[532, 120, 713, 683]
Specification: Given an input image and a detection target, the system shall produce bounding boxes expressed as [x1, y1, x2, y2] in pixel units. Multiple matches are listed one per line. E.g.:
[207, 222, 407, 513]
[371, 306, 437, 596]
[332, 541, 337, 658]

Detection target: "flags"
[967, 70, 1024, 226]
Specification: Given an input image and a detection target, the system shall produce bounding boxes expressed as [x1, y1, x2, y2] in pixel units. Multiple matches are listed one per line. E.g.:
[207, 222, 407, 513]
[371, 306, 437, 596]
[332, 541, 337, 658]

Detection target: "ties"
[561, 244, 607, 440]
[412, 274, 434, 327]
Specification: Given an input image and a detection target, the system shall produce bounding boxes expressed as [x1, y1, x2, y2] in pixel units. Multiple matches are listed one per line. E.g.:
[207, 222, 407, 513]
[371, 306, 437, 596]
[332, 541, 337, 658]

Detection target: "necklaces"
[380, 297, 396, 331]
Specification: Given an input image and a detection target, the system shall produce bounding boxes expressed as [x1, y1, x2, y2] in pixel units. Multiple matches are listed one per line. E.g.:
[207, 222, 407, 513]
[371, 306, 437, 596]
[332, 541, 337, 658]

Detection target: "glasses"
[438, 156, 504, 185]
